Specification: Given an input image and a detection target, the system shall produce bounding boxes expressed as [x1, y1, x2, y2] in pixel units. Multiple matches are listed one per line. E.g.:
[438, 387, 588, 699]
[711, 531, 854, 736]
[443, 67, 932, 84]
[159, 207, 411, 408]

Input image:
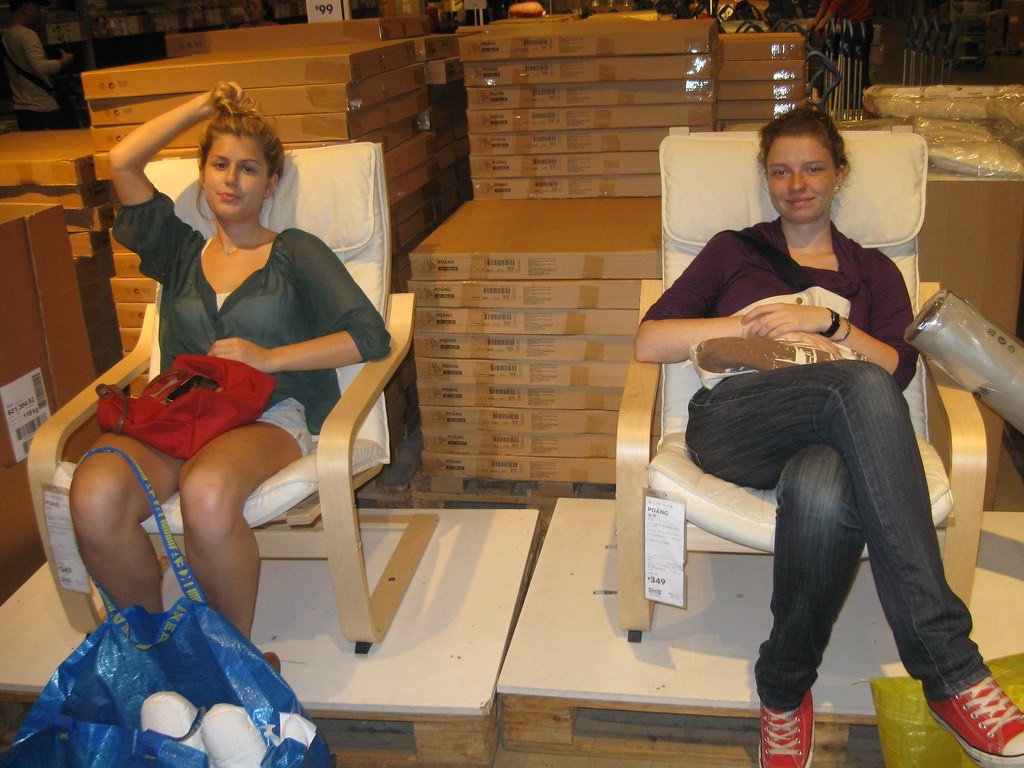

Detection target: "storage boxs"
[0, 0, 1024, 483]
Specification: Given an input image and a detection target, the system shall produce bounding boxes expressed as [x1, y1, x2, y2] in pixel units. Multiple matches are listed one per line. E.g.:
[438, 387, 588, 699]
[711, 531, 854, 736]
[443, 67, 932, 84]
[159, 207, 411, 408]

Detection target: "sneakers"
[927, 675, 1024, 768]
[758, 688, 815, 768]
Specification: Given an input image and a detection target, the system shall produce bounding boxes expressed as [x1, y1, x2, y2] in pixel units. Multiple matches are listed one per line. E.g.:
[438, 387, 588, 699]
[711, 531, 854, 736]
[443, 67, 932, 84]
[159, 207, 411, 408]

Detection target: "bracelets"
[832, 317, 851, 342]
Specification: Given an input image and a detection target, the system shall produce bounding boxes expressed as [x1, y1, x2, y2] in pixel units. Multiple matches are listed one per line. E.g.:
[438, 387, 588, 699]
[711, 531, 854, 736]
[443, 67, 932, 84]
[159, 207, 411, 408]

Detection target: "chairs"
[32, 139, 438, 648]
[613, 133, 991, 656]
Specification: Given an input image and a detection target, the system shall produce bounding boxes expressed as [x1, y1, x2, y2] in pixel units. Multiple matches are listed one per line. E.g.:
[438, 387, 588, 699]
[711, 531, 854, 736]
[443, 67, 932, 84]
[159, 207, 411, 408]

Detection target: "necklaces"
[216, 233, 261, 255]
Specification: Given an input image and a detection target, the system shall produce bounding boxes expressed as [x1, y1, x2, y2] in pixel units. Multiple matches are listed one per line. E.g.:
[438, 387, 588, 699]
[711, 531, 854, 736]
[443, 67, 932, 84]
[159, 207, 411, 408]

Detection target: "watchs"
[821, 307, 842, 339]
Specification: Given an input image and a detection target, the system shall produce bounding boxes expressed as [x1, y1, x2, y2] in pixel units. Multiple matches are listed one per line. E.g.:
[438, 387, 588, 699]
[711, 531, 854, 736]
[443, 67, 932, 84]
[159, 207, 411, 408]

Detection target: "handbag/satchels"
[96, 354, 278, 459]
[691, 227, 873, 390]
[0, 447, 336, 768]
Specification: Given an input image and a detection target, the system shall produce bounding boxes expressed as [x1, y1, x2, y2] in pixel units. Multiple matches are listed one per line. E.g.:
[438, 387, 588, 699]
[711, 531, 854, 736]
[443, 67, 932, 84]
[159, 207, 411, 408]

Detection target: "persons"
[70, 83, 392, 676]
[808, 0, 873, 107]
[0, 0, 75, 131]
[635, 108, 1024, 768]
[632, 0, 755, 20]
[238, 0, 277, 28]
